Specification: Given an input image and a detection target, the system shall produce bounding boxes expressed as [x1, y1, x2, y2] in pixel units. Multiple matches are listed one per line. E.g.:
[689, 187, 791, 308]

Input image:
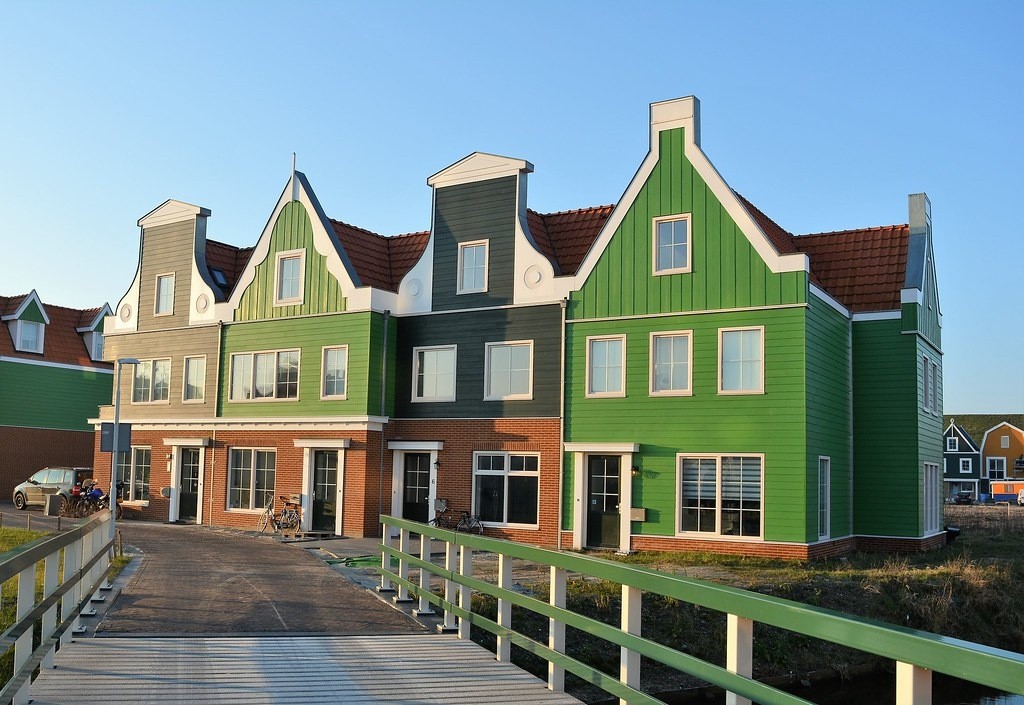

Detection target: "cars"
[954, 491, 972, 505]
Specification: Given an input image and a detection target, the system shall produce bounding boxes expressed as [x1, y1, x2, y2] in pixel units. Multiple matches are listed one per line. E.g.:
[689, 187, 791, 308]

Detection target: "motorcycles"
[77, 480, 125, 520]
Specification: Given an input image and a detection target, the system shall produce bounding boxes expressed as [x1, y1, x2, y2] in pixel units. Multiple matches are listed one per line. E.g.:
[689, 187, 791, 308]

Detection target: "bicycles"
[425, 498, 484, 541]
[257, 490, 300, 536]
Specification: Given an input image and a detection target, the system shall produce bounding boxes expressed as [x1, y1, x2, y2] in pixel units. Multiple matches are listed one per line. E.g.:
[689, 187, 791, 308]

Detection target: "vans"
[1016, 489, 1024, 506]
[11, 466, 94, 510]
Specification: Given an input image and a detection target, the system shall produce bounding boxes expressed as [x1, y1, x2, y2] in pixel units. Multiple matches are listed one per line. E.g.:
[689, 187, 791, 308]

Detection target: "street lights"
[108, 357, 141, 539]
